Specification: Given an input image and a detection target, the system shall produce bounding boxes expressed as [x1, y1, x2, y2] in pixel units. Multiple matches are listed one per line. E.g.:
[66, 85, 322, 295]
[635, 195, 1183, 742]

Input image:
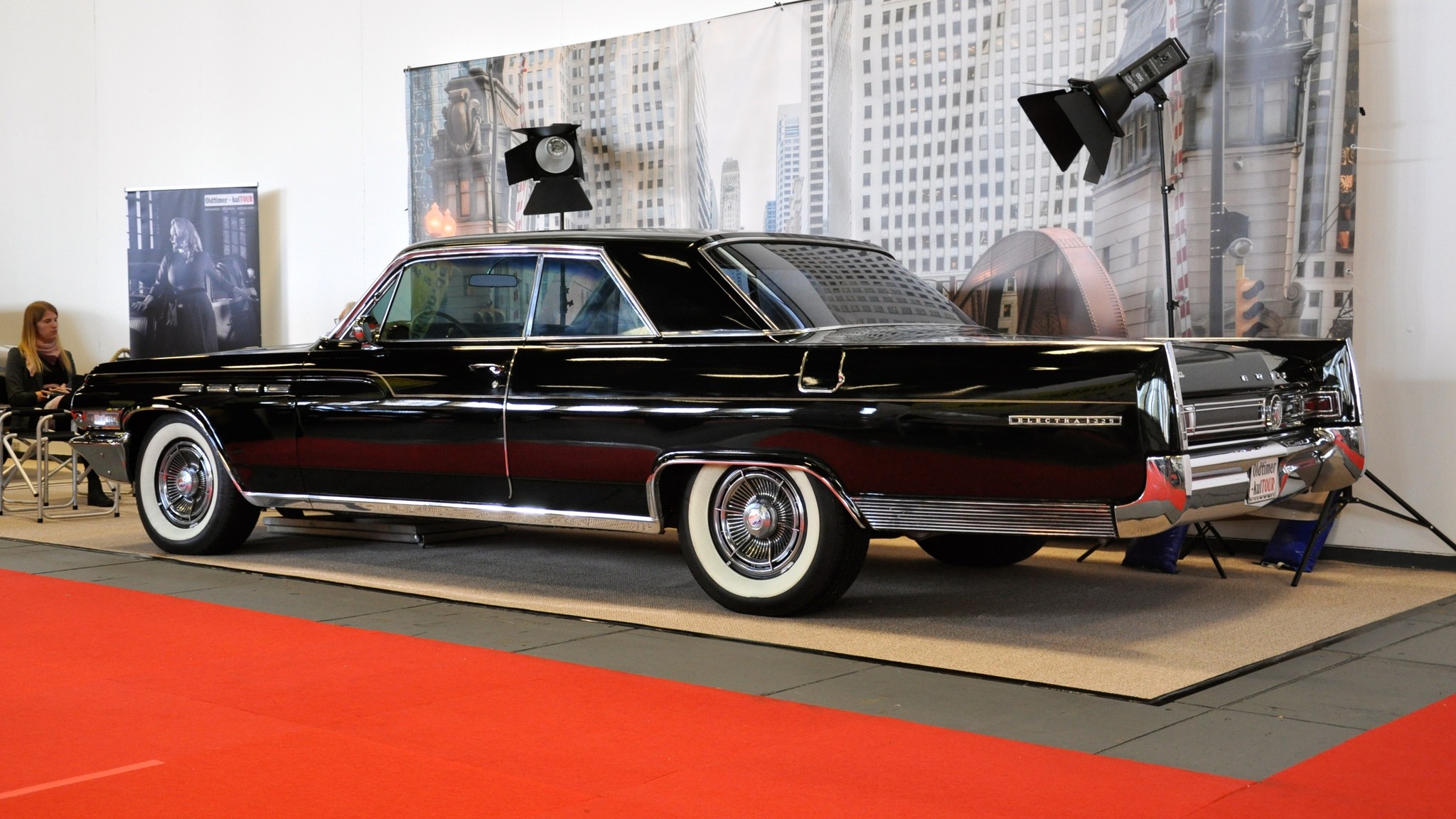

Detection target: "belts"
[177, 287, 206, 297]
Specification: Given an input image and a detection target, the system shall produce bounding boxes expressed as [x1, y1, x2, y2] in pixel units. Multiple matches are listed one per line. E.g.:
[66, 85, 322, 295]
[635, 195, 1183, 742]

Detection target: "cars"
[68, 226, 1366, 621]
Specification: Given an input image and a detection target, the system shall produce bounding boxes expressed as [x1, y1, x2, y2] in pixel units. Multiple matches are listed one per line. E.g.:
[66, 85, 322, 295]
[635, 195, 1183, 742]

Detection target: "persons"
[131, 217, 258, 356]
[5, 301, 115, 507]
[334, 301, 375, 325]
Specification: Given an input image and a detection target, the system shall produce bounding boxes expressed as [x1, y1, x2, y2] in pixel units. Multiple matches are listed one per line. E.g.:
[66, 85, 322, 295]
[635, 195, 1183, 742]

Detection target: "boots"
[84, 469, 114, 507]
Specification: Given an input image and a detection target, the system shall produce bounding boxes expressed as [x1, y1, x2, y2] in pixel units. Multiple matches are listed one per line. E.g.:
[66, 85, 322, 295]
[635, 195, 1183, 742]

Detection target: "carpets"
[1, 504, 1456, 702]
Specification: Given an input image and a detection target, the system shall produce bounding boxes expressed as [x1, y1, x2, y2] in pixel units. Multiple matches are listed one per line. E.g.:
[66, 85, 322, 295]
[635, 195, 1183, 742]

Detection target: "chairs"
[0, 344, 142, 524]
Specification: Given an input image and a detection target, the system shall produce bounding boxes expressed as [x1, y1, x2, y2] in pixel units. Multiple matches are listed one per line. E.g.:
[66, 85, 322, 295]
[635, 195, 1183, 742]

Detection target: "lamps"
[1017, 38, 1233, 579]
[502, 123, 593, 334]
